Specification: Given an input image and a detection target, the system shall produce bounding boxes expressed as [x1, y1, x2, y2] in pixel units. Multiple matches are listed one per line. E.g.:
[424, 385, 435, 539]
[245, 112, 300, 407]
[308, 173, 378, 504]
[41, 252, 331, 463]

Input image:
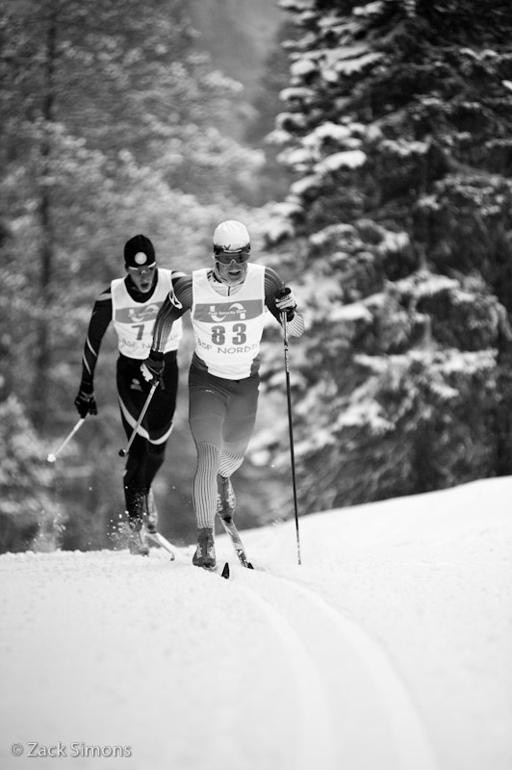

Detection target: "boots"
[126, 516, 152, 555]
[217, 474, 236, 516]
[140, 485, 160, 534]
[192, 526, 217, 567]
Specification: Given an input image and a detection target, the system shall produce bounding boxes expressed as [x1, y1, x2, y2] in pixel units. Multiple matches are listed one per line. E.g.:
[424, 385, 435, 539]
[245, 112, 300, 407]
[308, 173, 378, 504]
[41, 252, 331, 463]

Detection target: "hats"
[124, 234, 156, 269]
[212, 220, 250, 252]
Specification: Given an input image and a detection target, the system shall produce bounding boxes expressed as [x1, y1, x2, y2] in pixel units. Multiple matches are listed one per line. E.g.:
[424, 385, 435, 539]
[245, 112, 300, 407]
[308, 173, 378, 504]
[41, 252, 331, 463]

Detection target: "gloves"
[74, 387, 97, 419]
[273, 287, 297, 313]
[138, 349, 167, 392]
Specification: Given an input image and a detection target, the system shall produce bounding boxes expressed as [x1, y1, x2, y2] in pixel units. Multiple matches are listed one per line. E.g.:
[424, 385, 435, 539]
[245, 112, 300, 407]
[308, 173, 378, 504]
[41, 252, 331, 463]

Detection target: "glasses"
[213, 253, 252, 265]
[127, 262, 157, 274]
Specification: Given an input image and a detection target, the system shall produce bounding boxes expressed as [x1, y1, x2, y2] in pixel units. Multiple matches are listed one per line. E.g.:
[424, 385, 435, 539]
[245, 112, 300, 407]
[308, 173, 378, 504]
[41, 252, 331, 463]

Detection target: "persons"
[74, 234, 188, 554]
[138, 220, 305, 566]
